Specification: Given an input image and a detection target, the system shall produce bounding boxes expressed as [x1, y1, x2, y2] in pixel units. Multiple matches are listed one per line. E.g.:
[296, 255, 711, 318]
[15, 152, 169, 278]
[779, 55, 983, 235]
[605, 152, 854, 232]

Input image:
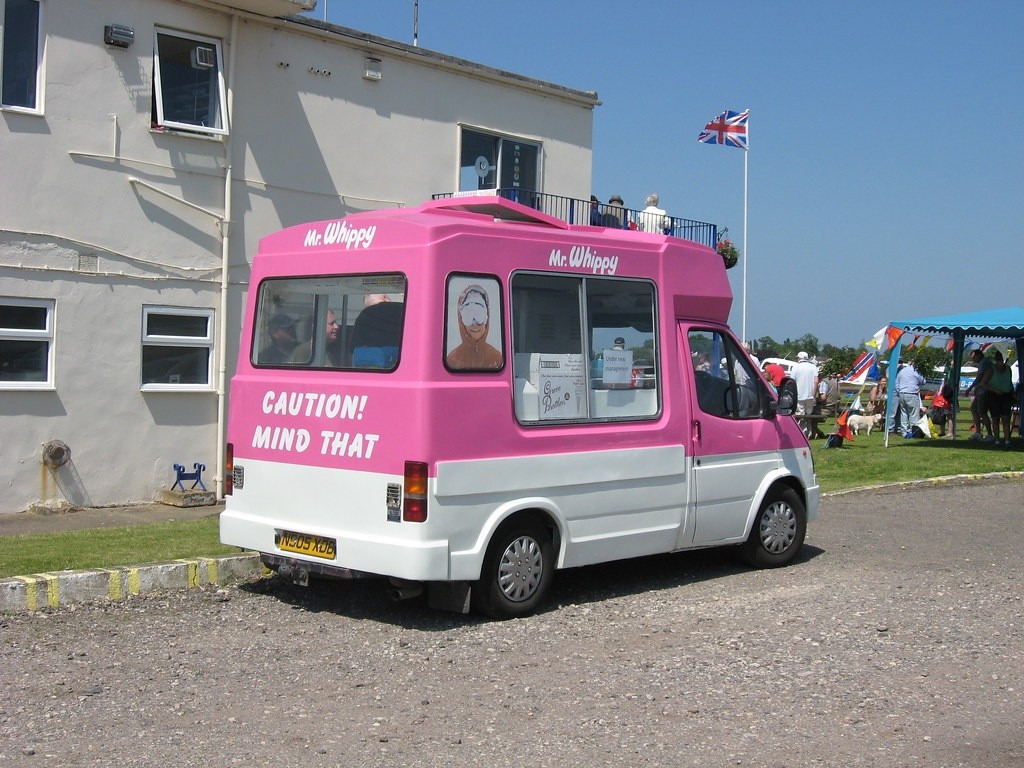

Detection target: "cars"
[760, 357, 798, 377]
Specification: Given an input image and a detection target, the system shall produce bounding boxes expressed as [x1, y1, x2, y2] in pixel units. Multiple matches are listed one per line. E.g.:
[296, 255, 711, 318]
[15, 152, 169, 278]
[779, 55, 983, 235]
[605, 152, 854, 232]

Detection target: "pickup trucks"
[217, 189, 821, 620]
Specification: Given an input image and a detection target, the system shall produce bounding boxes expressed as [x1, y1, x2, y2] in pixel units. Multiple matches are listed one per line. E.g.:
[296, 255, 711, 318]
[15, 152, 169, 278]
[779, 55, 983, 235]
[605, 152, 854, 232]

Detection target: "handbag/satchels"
[912, 394, 950, 438]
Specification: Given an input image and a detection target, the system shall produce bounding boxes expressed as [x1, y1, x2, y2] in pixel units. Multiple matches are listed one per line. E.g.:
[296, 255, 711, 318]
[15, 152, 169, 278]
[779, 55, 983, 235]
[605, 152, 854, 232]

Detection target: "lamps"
[103, 23, 136, 50]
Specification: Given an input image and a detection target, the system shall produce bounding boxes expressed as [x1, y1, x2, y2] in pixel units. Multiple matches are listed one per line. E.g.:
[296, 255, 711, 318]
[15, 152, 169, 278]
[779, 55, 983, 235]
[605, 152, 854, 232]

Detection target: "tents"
[884, 305, 1024, 447]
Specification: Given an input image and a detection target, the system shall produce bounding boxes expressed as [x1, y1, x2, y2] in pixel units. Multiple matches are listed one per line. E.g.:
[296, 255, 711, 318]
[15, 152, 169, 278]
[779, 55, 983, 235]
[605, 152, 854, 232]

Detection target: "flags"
[880, 325, 996, 355]
[697, 109, 750, 148]
[837, 350, 876, 382]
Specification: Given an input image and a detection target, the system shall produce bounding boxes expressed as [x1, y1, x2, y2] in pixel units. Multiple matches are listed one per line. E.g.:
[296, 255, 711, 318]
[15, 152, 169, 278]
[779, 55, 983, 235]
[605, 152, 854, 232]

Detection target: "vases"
[724, 257, 738, 269]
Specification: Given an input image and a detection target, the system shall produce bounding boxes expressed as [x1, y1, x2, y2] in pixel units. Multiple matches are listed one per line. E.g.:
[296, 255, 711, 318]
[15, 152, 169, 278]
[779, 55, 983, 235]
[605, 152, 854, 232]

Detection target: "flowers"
[716, 239, 743, 260]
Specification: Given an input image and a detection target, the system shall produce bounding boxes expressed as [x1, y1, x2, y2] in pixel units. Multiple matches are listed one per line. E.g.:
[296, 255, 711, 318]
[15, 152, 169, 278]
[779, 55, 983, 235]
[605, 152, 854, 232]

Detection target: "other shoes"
[941, 433, 953, 439]
[1005, 440, 1010, 445]
[969, 432, 982, 440]
[981, 434, 994, 441]
[995, 441, 1000, 446]
[808, 434, 815, 439]
[816, 432, 824, 440]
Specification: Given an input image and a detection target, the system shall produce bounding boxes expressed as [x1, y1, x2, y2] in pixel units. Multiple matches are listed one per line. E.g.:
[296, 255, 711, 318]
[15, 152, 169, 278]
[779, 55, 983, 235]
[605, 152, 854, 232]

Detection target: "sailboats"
[835, 339, 880, 394]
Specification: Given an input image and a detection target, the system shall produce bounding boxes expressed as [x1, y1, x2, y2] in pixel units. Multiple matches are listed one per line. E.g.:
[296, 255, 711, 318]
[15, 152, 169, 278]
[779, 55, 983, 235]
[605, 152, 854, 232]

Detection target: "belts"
[899, 392, 918, 395]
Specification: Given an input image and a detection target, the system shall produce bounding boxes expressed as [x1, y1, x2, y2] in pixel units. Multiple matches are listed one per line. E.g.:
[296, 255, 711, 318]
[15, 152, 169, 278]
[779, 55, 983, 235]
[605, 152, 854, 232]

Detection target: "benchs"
[795, 415, 828, 439]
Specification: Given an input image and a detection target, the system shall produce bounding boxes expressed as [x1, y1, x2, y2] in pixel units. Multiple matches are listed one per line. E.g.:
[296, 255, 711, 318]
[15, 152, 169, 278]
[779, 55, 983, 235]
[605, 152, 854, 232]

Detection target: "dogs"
[847, 414, 882, 437]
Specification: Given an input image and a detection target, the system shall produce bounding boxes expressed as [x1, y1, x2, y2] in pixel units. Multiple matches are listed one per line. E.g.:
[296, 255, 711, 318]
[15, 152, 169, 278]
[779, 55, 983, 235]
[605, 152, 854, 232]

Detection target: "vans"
[632, 365, 655, 378]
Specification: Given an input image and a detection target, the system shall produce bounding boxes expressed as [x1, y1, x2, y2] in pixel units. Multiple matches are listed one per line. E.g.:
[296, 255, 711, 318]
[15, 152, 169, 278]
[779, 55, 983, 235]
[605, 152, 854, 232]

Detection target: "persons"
[965, 349, 994, 442]
[895, 364, 926, 437]
[638, 192, 680, 236]
[817, 373, 841, 418]
[762, 362, 785, 393]
[789, 351, 819, 416]
[720, 358, 729, 381]
[611, 336, 626, 351]
[696, 352, 712, 374]
[734, 341, 761, 387]
[285, 307, 340, 367]
[982, 350, 1015, 446]
[256, 313, 304, 364]
[601, 194, 635, 230]
[937, 358, 954, 437]
[870, 376, 888, 430]
[363, 291, 391, 306]
[590, 194, 602, 227]
[888, 359, 910, 433]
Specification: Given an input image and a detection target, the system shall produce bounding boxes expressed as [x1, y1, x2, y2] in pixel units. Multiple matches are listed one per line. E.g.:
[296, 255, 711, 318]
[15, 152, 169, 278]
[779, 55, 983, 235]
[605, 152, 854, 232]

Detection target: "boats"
[915, 364, 979, 400]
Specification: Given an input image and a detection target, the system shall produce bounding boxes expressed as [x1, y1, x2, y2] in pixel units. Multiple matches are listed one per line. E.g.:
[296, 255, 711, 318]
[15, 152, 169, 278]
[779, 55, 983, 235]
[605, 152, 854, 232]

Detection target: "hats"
[267, 313, 298, 331]
[796, 351, 808, 360]
[608, 195, 624, 205]
[591, 195, 602, 204]
[614, 337, 625, 344]
